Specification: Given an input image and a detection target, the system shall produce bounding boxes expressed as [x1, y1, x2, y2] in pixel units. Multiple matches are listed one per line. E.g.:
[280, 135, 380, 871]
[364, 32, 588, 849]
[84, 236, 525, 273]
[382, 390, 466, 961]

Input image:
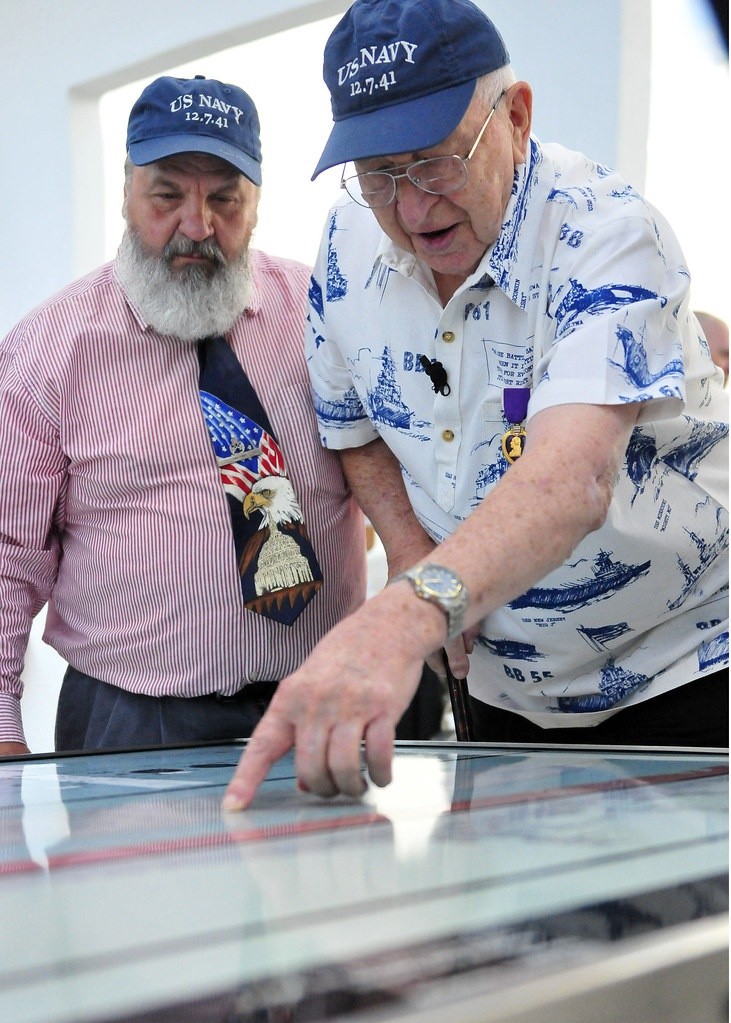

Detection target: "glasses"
[341, 91, 507, 209]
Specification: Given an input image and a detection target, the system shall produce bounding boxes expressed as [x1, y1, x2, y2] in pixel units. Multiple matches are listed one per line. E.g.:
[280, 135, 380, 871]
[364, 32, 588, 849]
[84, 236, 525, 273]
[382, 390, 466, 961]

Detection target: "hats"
[311, 0, 510, 181]
[126, 75, 265, 187]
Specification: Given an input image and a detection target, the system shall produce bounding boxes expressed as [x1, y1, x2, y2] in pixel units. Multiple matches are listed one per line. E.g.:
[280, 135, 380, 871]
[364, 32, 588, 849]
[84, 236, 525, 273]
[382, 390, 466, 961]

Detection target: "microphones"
[418, 353, 451, 396]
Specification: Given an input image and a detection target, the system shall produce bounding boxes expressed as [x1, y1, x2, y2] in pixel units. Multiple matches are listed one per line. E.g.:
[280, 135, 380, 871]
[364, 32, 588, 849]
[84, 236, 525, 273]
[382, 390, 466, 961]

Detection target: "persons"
[0, 75, 369, 755]
[221, 0, 729, 812]
[692, 310, 729, 388]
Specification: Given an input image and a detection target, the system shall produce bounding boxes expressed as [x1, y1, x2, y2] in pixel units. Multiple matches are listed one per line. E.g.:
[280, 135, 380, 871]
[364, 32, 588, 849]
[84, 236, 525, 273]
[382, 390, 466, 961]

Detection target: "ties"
[196, 333, 323, 626]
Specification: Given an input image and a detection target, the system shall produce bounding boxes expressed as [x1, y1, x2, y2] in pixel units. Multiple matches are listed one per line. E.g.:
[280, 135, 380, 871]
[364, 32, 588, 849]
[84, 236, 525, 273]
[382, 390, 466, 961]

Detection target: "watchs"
[394, 563, 468, 641]
